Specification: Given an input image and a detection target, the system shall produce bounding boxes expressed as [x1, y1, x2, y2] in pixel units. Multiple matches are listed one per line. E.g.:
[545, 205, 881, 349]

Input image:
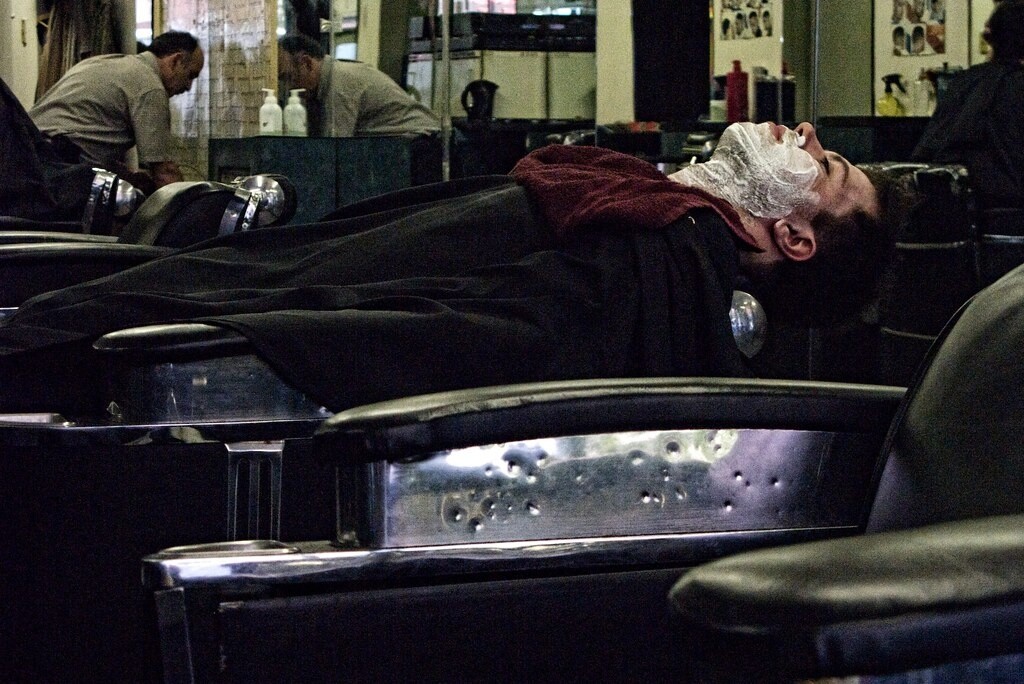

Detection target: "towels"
[506, 142, 768, 254]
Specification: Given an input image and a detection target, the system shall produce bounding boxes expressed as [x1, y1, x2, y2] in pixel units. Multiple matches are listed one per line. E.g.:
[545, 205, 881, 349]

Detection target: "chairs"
[0, 72, 1024, 684]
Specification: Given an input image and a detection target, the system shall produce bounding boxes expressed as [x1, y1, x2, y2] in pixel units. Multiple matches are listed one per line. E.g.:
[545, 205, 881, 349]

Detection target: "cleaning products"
[877, 73, 906, 117]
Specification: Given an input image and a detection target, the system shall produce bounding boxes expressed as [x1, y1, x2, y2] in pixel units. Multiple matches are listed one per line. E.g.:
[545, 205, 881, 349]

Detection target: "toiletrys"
[258, 88, 283, 137]
[710, 59, 749, 122]
[284, 88, 307, 138]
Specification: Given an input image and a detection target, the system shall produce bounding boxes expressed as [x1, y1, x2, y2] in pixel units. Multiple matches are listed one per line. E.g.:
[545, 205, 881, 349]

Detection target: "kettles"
[461, 79, 499, 118]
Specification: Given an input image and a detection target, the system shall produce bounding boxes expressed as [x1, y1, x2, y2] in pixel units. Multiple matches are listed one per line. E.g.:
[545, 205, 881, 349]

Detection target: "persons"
[664, 121, 904, 301]
[26, 30, 203, 197]
[720, 0, 1024, 210]
[277, 32, 485, 184]
